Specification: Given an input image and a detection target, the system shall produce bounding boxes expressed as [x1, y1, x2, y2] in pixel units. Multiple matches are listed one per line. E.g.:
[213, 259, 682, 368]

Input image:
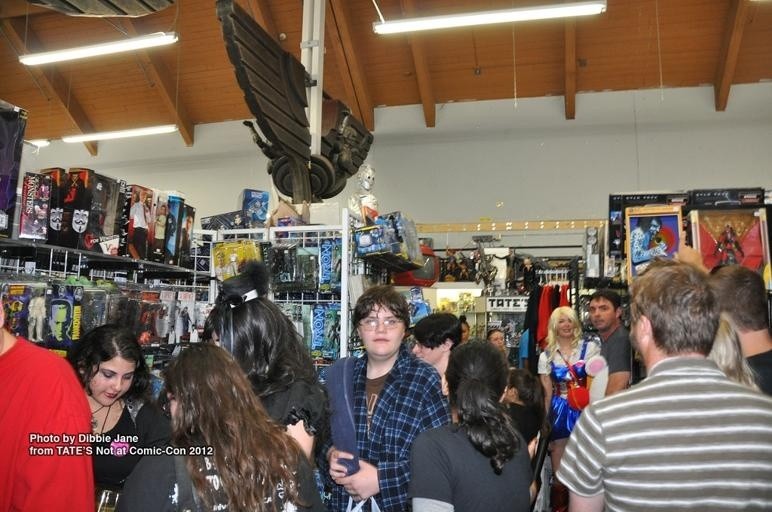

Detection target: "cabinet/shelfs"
[217, 208, 394, 361]
[401, 278, 476, 343]
[2, 228, 215, 422]
[479, 295, 529, 364]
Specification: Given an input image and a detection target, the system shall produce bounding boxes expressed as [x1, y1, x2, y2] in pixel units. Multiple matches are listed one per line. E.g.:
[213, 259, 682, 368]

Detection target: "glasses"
[160, 396, 177, 419]
[356, 319, 404, 330]
[411, 340, 424, 349]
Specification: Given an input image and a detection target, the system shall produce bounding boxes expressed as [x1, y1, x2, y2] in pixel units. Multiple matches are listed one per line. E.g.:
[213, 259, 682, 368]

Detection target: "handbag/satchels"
[556, 347, 589, 411]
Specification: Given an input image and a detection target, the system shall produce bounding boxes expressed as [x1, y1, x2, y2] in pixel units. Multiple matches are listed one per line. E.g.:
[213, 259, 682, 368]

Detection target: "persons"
[504, 368, 544, 446]
[203, 297, 324, 461]
[0, 303, 95, 512]
[710, 220, 746, 264]
[413, 313, 462, 400]
[180, 214, 193, 256]
[631, 217, 679, 261]
[66, 325, 174, 512]
[714, 265, 772, 399]
[115, 344, 328, 512]
[409, 340, 536, 512]
[446, 318, 510, 357]
[556, 261, 772, 512]
[707, 318, 761, 387]
[538, 306, 601, 512]
[153, 204, 168, 260]
[590, 291, 631, 395]
[318, 288, 454, 512]
[128, 186, 153, 259]
[26, 288, 46, 343]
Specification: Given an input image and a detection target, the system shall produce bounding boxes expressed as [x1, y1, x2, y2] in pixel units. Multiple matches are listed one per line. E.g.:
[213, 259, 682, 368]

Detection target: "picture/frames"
[624, 203, 682, 285]
[692, 208, 771, 292]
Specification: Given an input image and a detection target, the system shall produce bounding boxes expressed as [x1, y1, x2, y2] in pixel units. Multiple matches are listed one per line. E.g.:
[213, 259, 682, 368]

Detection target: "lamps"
[373, 1, 607, 34]
[62, 124, 180, 144]
[26, 139, 51, 147]
[17, 32, 179, 66]
